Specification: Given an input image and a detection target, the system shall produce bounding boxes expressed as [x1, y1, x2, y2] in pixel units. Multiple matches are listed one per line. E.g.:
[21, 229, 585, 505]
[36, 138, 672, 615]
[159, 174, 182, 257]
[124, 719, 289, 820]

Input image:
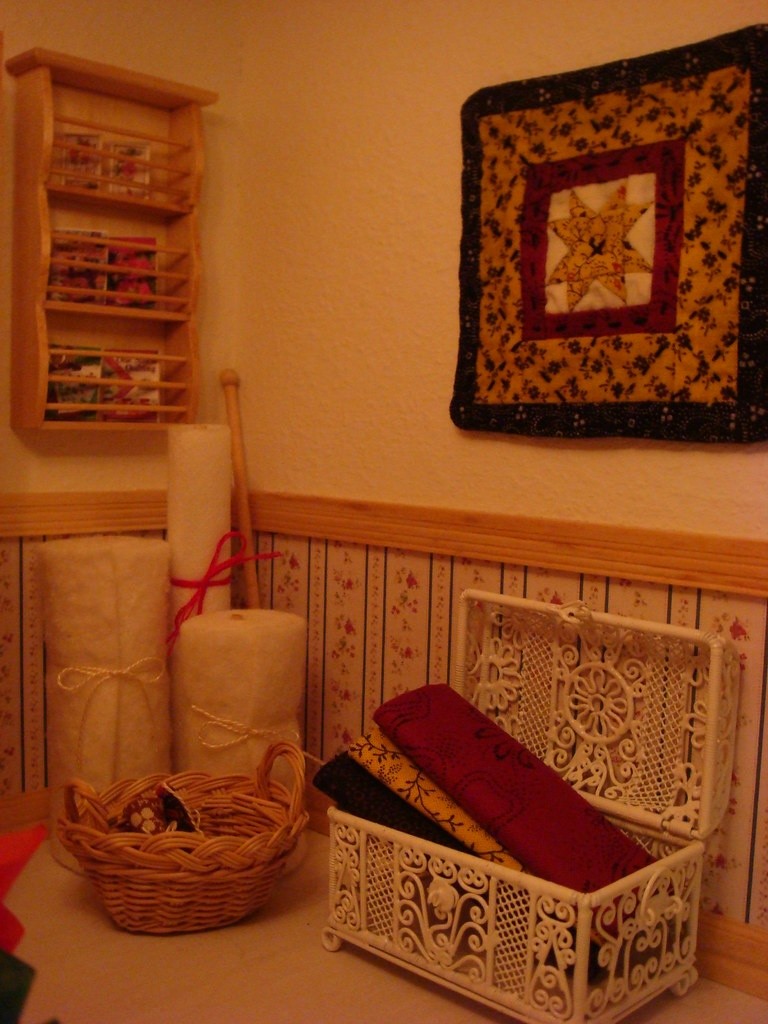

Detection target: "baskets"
[56, 740, 309, 934]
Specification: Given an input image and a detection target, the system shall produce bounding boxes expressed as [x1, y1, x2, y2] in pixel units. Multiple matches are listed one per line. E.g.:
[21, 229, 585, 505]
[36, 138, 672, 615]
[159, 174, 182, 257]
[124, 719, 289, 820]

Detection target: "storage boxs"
[323, 589, 740, 1024]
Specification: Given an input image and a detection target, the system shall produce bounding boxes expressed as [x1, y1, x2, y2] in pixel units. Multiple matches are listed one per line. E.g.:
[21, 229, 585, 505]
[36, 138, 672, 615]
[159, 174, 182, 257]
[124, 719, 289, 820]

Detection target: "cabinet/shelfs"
[6, 47, 220, 431]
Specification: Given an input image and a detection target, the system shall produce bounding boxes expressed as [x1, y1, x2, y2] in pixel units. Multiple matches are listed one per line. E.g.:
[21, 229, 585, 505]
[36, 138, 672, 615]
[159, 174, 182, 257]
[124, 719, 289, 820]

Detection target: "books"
[49, 228, 160, 302]
[48, 347, 163, 416]
